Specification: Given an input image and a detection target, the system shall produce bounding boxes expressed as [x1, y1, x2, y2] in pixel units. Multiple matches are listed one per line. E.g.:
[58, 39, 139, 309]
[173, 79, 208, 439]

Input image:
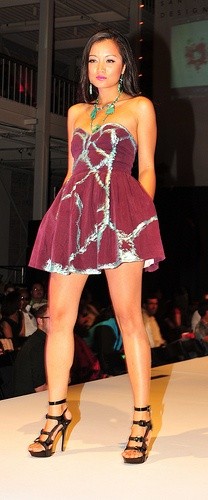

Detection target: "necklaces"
[90, 88, 122, 133]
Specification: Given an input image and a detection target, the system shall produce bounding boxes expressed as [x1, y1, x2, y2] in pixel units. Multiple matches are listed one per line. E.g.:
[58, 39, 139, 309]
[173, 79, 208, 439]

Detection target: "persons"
[0, 282, 86, 401]
[77, 291, 208, 381]
[27, 29, 164, 463]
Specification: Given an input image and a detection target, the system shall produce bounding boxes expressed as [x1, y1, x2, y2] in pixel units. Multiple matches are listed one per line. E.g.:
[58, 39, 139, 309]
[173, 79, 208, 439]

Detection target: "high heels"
[30, 399, 72, 457]
[123, 407, 152, 463]
